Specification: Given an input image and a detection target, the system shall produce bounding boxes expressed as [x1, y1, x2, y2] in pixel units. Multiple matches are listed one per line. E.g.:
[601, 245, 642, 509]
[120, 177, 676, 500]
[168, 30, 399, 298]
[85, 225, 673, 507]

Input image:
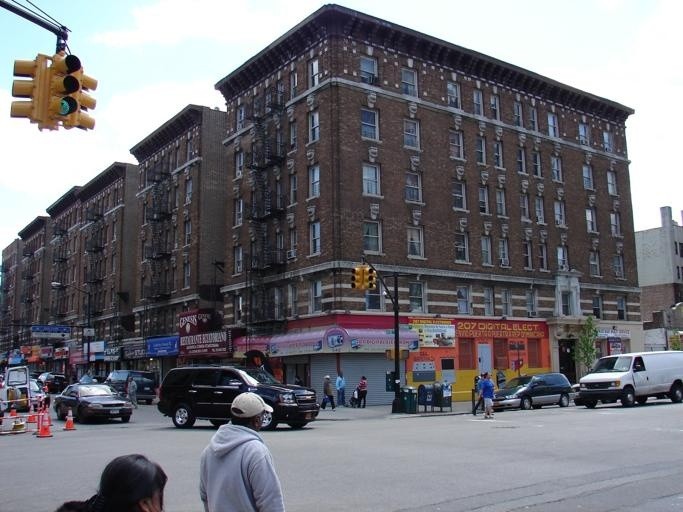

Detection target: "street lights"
[49, 282, 91, 372]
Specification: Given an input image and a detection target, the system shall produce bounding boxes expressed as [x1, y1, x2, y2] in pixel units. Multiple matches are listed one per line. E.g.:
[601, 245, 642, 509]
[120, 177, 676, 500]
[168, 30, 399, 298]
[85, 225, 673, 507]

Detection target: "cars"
[53, 385, 133, 423]
[30, 380, 50, 412]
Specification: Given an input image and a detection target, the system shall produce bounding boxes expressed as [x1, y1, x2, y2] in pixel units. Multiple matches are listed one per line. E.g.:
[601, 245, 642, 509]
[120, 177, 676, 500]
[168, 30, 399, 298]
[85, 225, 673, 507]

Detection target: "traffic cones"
[33, 410, 42, 435]
[39, 413, 52, 437]
[63, 407, 76, 431]
[44, 405, 53, 426]
[26, 405, 37, 423]
[10, 405, 17, 417]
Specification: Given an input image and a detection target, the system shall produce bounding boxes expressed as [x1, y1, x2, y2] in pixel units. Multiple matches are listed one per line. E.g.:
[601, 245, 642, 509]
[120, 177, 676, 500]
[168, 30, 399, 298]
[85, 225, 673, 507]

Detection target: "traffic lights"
[12, 57, 97, 129]
[351, 264, 377, 291]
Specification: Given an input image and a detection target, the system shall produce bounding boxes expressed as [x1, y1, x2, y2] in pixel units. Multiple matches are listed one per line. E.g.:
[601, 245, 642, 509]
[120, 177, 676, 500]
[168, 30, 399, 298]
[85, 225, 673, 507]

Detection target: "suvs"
[158, 366, 320, 430]
[491, 373, 576, 411]
[35, 372, 68, 394]
[104, 370, 156, 403]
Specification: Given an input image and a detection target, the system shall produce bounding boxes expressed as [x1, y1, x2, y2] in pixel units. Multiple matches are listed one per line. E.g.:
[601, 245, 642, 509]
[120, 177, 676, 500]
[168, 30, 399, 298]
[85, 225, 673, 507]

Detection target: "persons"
[479, 373, 494, 419]
[79, 368, 93, 385]
[56, 455, 167, 511]
[126, 377, 139, 409]
[358, 376, 368, 408]
[473, 373, 484, 416]
[295, 376, 303, 387]
[336, 371, 346, 406]
[89, 370, 96, 379]
[321, 375, 336, 411]
[200, 392, 285, 511]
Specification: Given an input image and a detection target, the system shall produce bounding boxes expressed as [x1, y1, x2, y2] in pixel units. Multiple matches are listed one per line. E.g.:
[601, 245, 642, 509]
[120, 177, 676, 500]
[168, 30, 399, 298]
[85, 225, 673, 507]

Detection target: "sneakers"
[330, 408, 336, 411]
[321, 405, 328, 410]
[484, 415, 489, 419]
[489, 415, 494, 419]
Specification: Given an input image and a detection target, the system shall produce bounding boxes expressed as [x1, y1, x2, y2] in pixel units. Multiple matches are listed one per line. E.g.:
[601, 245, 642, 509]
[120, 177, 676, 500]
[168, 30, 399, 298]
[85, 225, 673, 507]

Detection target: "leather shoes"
[472, 409, 477, 416]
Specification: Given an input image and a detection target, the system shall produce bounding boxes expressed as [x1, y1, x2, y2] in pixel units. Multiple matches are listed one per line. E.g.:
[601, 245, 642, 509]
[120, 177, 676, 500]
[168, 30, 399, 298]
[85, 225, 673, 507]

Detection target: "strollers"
[349, 387, 362, 407]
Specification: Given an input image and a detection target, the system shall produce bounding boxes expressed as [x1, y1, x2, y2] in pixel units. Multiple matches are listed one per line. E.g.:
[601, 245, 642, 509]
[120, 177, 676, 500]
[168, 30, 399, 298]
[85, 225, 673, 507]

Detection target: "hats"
[323, 375, 330, 379]
[230, 392, 275, 418]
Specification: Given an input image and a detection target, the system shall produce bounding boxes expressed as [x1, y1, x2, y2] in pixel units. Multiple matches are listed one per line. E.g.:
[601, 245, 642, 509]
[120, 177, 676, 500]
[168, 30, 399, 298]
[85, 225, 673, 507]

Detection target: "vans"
[579, 351, 683, 408]
